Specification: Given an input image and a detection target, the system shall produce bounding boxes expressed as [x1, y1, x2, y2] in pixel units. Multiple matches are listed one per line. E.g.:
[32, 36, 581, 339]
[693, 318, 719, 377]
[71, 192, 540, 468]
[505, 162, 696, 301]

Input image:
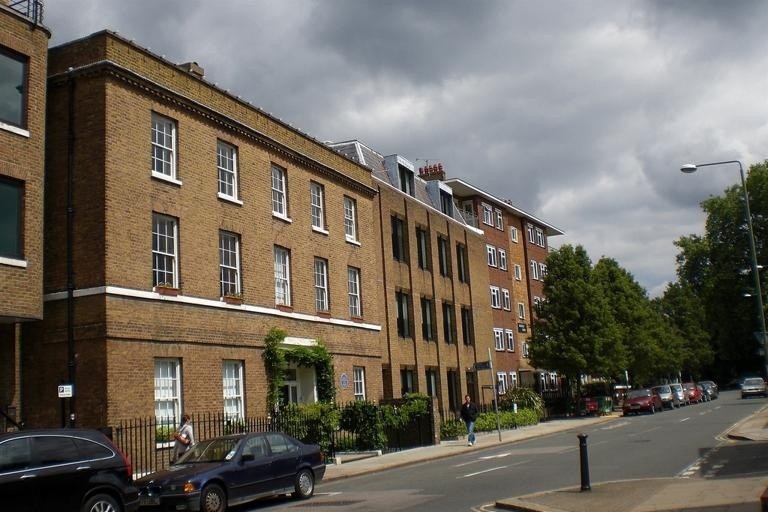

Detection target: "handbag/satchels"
[173, 431, 191, 446]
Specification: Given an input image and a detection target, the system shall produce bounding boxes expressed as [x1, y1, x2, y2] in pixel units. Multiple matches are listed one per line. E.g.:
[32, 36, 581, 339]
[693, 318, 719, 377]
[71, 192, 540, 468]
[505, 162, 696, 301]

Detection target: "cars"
[739, 376, 768, 399]
[623, 380, 718, 415]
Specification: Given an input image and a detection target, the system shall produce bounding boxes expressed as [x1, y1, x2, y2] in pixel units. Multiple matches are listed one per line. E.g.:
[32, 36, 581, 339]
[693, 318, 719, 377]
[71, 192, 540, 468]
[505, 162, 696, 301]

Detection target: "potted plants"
[351, 315, 364, 323]
[155, 281, 180, 296]
[317, 311, 331, 318]
[276, 303, 294, 313]
[224, 292, 244, 305]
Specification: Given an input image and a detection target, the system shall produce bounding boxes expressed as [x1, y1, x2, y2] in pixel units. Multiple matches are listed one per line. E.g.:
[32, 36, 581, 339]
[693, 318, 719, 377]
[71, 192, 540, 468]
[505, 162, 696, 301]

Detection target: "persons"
[173, 415, 194, 464]
[460, 395, 479, 446]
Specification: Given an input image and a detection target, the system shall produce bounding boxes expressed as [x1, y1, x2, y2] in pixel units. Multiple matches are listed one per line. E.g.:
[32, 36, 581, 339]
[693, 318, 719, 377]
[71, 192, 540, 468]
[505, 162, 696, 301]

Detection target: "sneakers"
[467, 440, 477, 447]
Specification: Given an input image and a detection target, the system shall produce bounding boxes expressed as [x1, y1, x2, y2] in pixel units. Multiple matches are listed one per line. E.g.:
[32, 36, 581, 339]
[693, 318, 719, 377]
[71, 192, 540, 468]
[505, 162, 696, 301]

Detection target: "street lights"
[681, 160, 768, 364]
